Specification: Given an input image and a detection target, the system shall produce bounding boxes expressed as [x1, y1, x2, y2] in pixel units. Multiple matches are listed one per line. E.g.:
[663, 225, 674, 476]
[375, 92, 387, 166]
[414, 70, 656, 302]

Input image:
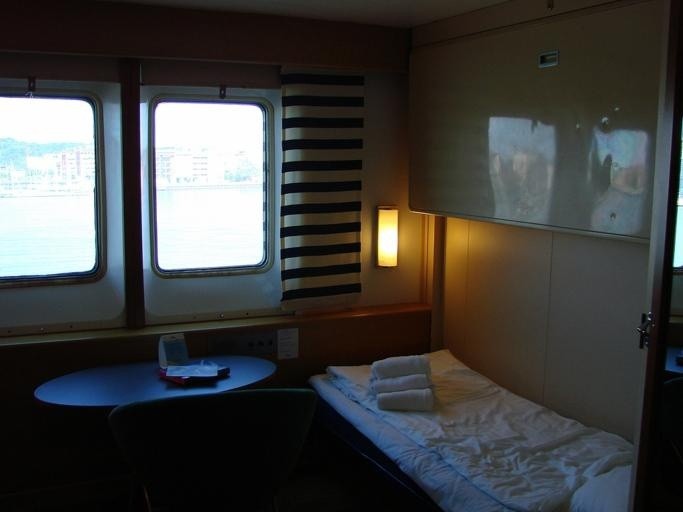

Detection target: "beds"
[310, 346, 633, 512]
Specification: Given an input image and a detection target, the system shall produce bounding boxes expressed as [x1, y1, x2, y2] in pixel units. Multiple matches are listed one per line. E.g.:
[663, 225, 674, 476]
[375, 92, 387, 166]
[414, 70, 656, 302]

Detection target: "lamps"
[377, 204, 401, 270]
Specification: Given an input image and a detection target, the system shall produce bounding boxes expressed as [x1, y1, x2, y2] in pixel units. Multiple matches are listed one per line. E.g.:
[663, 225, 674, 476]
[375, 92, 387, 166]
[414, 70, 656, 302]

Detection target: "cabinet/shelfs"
[405, 0, 672, 245]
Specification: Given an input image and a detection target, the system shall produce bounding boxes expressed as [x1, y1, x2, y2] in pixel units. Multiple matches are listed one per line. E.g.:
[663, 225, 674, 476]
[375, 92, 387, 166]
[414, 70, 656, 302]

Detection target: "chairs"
[109, 387, 316, 512]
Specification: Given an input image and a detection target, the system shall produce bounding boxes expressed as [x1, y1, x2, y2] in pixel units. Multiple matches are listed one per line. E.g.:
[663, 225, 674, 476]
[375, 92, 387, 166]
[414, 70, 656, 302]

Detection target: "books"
[160, 359, 231, 388]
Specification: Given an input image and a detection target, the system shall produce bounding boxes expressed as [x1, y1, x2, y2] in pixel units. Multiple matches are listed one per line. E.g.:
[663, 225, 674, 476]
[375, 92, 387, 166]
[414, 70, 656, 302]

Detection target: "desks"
[30, 353, 277, 500]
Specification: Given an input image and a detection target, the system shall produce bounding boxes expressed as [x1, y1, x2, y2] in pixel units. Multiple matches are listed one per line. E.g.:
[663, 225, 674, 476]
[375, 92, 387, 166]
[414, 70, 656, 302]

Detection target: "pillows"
[569, 463, 632, 512]
